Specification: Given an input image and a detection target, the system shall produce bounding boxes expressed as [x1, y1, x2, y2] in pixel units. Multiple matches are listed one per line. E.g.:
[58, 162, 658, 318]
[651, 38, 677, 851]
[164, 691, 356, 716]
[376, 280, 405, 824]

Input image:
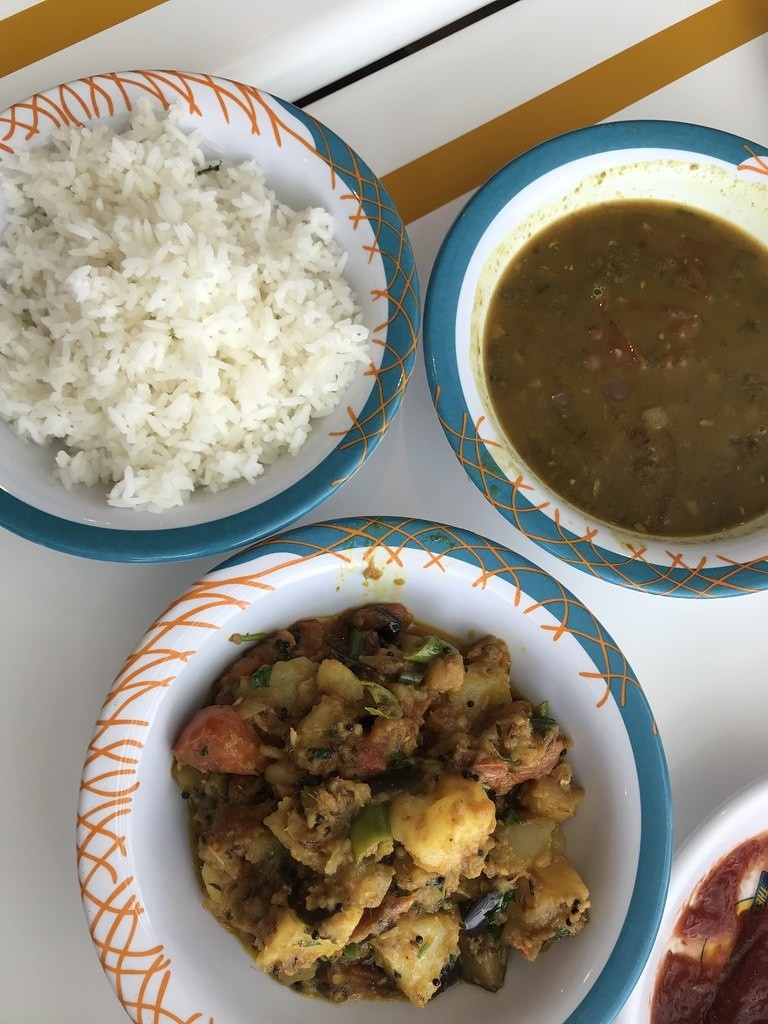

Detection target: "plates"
[76, 515, 673, 1024]
[611, 773, 768, 1024]
[421, 117, 768, 599]
[0, 69, 420, 564]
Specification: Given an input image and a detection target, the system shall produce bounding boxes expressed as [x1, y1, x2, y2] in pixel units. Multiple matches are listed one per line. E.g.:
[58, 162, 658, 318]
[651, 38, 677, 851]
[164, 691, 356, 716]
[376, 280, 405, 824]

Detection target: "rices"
[0, 95, 376, 516]
